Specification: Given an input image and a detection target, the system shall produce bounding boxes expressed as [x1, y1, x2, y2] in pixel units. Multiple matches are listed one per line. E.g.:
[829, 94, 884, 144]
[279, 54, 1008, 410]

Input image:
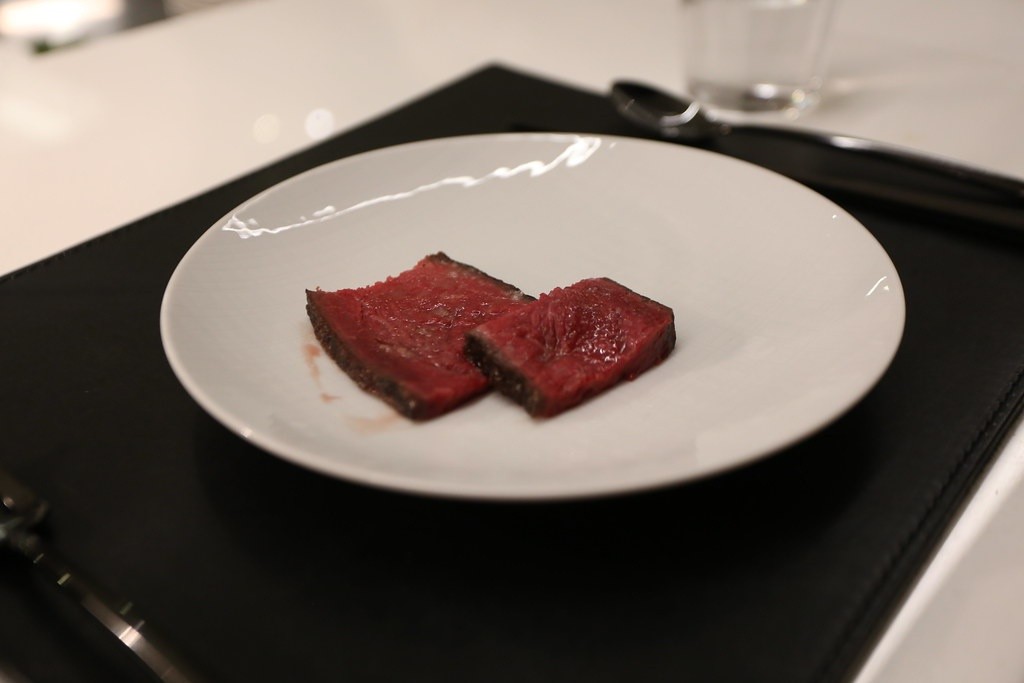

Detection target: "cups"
[681, 2, 831, 128]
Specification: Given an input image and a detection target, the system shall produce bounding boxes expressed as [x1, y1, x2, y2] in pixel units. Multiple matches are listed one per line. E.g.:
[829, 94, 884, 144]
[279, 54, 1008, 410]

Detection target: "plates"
[158, 132, 910, 502]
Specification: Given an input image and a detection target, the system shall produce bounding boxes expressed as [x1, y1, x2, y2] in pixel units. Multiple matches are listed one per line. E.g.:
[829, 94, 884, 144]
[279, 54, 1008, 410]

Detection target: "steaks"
[305, 252, 678, 420]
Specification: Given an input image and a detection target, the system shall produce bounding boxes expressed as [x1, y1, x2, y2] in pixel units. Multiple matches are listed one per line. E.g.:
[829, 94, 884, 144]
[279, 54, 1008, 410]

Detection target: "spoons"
[610, 76, 1021, 242]
